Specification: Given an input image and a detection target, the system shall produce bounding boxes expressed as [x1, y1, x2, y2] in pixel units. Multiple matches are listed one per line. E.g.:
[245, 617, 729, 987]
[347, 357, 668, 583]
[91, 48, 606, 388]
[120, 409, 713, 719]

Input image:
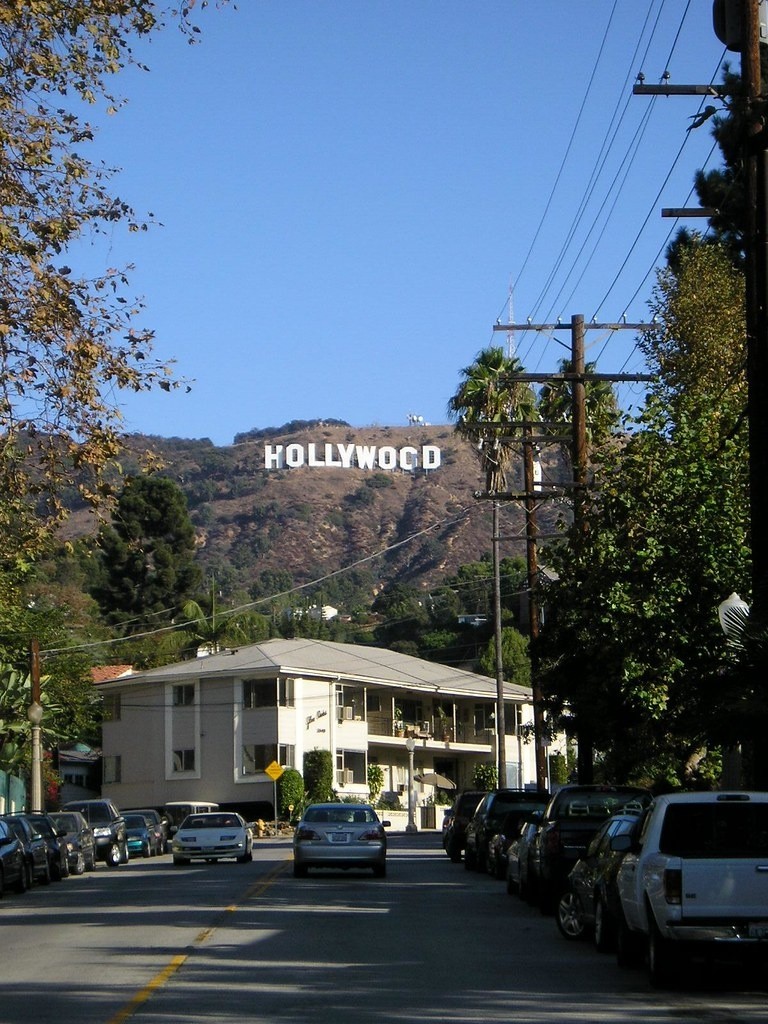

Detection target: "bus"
[163, 801, 219, 834]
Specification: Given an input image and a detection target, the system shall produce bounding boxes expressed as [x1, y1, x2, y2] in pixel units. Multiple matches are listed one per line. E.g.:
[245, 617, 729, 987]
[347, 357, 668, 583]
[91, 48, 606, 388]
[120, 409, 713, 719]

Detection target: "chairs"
[315, 810, 327, 821]
[353, 812, 366, 822]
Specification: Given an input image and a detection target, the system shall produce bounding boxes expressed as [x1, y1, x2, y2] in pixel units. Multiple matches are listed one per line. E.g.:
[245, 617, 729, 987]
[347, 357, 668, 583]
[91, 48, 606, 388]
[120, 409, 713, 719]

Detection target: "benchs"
[405, 724, 432, 739]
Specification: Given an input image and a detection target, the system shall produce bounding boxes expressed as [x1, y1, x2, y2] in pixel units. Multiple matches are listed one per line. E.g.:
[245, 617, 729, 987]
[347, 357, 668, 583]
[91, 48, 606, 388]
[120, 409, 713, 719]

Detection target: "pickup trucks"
[606, 790, 768, 987]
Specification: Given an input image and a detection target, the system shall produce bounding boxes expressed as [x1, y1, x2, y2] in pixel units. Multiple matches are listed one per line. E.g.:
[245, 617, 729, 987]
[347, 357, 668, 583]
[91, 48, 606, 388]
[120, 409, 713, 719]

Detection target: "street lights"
[28, 700, 46, 814]
[405, 734, 419, 834]
[716, 588, 749, 792]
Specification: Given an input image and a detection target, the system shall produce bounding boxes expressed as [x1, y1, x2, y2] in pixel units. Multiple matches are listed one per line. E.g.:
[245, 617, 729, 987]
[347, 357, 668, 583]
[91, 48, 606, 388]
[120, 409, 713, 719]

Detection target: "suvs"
[441, 785, 656, 916]
[56, 797, 129, 867]
[119, 808, 169, 856]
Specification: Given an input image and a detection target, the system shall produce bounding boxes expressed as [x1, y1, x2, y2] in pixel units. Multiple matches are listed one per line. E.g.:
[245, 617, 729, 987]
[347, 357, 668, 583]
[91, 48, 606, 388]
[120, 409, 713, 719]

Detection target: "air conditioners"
[339, 706, 352, 719]
[338, 771, 354, 783]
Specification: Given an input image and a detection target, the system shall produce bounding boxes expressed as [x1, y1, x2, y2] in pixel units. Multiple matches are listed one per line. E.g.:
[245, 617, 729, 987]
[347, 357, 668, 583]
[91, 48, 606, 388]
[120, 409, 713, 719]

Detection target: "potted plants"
[394, 708, 405, 737]
[420, 791, 453, 831]
[438, 707, 452, 742]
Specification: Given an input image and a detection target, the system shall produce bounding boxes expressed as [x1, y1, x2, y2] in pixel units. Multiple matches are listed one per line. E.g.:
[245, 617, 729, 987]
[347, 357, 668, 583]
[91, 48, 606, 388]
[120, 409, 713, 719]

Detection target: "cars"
[121, 813, 155, 859]
[290, 802, 393, 878]
[555, 806, 645, 951]
[1, 808, 70, 882]
[0, 814, 54, 887]
[0, 816, 28, 897]
[168, 812, 256, 866]
[46, 811, 96, 874]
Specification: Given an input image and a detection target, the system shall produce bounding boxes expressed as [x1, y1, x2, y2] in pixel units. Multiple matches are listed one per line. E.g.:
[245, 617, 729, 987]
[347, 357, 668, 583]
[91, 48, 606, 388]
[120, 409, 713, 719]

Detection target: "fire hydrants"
[256, 819, 266, 837]
